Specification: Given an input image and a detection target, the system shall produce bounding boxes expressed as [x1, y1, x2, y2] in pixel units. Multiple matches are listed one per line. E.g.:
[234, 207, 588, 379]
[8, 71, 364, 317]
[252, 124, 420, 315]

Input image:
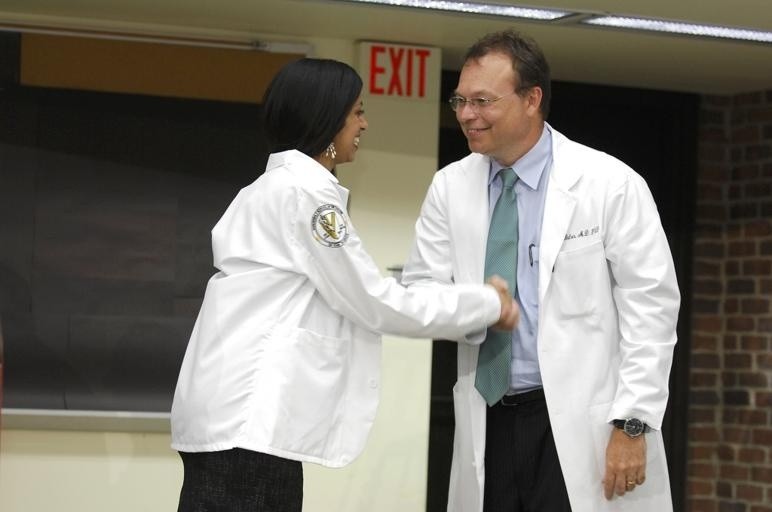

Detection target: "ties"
[474, 168, 518, 408]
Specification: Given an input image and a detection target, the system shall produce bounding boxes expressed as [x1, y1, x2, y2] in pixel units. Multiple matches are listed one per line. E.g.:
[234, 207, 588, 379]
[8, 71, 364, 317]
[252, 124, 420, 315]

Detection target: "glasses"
[448, 90, 514, 112]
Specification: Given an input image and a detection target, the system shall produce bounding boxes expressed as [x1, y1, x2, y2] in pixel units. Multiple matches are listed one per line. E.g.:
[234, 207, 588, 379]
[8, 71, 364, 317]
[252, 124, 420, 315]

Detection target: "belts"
[486, 388, 545, 408]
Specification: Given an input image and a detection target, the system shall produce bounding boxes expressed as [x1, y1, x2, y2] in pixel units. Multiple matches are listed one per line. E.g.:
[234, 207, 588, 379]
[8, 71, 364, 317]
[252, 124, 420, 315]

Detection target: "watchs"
[613, 417, 647, 437]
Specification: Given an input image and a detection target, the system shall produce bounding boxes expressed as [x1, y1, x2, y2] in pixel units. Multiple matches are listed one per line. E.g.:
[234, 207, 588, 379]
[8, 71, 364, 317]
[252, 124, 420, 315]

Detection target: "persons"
[401, 28, 681, 512]
